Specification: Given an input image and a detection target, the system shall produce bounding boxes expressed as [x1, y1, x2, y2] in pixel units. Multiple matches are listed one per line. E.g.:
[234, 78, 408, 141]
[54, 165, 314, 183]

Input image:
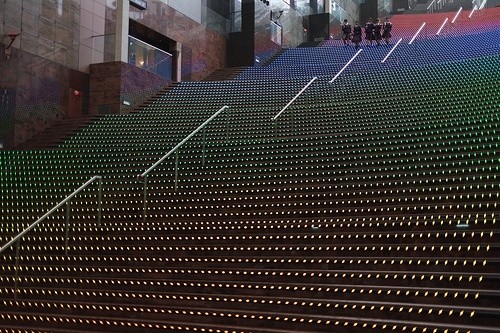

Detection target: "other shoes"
[343, 41, 390, 50]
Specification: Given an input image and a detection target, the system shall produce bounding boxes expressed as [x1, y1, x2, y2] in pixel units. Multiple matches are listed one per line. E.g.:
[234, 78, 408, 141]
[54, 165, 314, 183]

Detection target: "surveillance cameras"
[8, 32, 20, 37]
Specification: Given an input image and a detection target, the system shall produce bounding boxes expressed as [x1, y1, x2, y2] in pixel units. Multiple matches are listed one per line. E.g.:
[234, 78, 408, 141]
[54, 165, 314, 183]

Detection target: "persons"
[382, 17, 393, 46]
[374, 17, 382, 46]
[342, 19, 350, 47]
[351, 20, 361, 47]
[364, 17, 375, 47]
